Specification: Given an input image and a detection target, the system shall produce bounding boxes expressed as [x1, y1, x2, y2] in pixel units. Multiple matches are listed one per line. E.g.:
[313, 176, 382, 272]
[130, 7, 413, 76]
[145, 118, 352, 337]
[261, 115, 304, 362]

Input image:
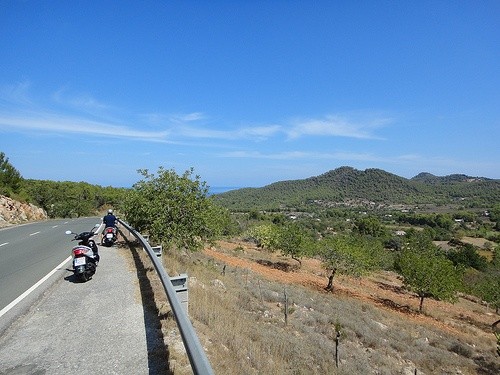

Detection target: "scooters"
[65, 224, 100, 282]
[101, 216, 120, 247]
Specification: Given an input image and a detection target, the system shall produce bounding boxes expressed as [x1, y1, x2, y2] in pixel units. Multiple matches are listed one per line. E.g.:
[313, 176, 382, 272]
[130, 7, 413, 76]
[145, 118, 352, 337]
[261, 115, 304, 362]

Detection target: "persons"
[100, 208, 119, 245]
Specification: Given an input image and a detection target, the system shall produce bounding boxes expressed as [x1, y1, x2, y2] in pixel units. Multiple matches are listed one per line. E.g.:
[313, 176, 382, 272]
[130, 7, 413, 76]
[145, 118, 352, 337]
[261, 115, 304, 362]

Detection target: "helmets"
[107, 208, 113, 214]
